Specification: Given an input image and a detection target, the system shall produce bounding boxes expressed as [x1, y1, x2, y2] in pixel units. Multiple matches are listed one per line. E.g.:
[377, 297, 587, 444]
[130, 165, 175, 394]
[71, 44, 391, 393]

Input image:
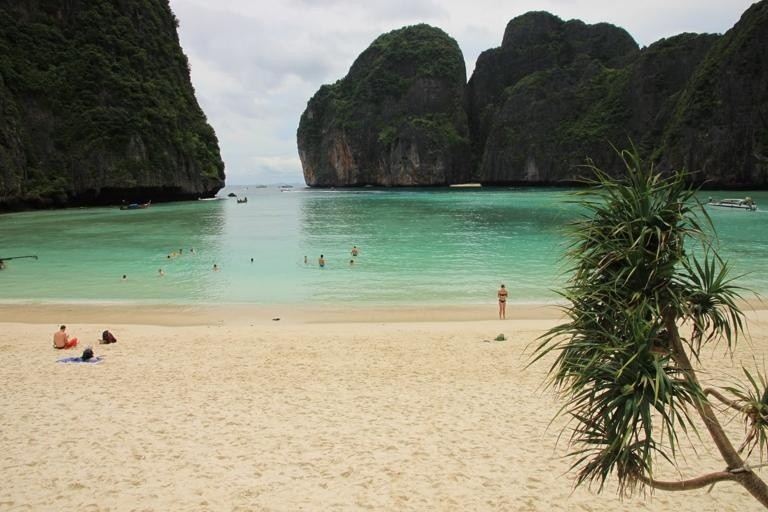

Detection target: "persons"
[55, 349, 104, 363]
[54, 325, 78, 349]
[497, 285, 508, 318]
[122, 245, 358, 279]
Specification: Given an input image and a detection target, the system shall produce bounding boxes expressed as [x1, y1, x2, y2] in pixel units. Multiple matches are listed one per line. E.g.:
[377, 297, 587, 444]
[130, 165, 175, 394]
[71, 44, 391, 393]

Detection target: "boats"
[120, 201, 150, 210]
[238, 197, 247, 203]
[707, 198, 757, 211]
